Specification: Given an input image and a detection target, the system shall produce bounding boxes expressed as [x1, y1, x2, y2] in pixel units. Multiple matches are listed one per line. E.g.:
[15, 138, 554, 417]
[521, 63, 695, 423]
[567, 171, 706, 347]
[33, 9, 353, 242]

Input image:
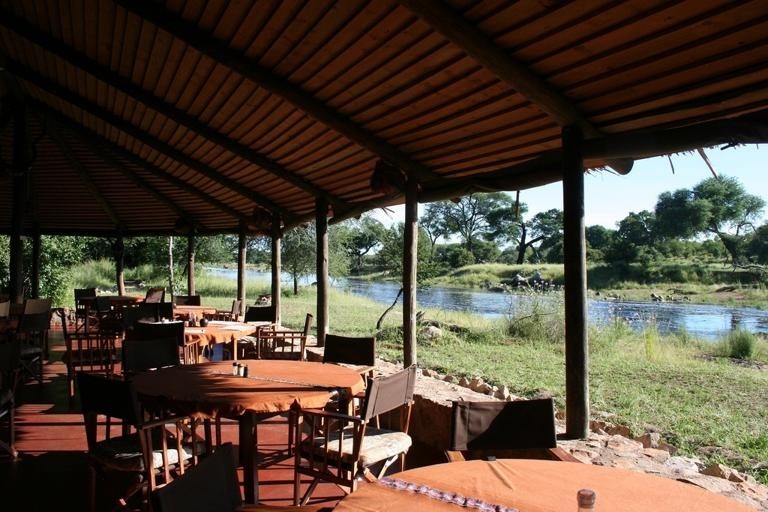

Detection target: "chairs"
[9, 298, 52, 346]
[143, 288, 164, 303]
[215, 300, 241, 322]
[123, 337, 180, 386]
[1, 327, 43, 385]
[74, 287, 96, 332]
[139, 408, 274, 512]
[442, 398, 583, 464]
[240, 409, 365, 511]
[256, 313, 312, 361]
[229, 334, 239, 360]
[159, 302, 196, 327]
[241, 303, 278, 356]
[183, 338, 221, 449]
[124, 307, 157, 337]
[132, 320, 200, 365]
[74, 367, 202, 512]
[60, 307, 119, 407]
[291, 364, 416, 508]
[0, 369, 20, 459]
[319, 334, 381, 429]
[173, 295, 201, 306]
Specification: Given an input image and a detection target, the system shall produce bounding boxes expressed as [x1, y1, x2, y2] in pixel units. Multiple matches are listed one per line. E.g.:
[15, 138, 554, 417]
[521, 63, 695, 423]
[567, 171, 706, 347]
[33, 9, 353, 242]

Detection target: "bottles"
[238, 364, 244, 376]
[577, 489, 596, 512]
[233, 362, 238, 375]
